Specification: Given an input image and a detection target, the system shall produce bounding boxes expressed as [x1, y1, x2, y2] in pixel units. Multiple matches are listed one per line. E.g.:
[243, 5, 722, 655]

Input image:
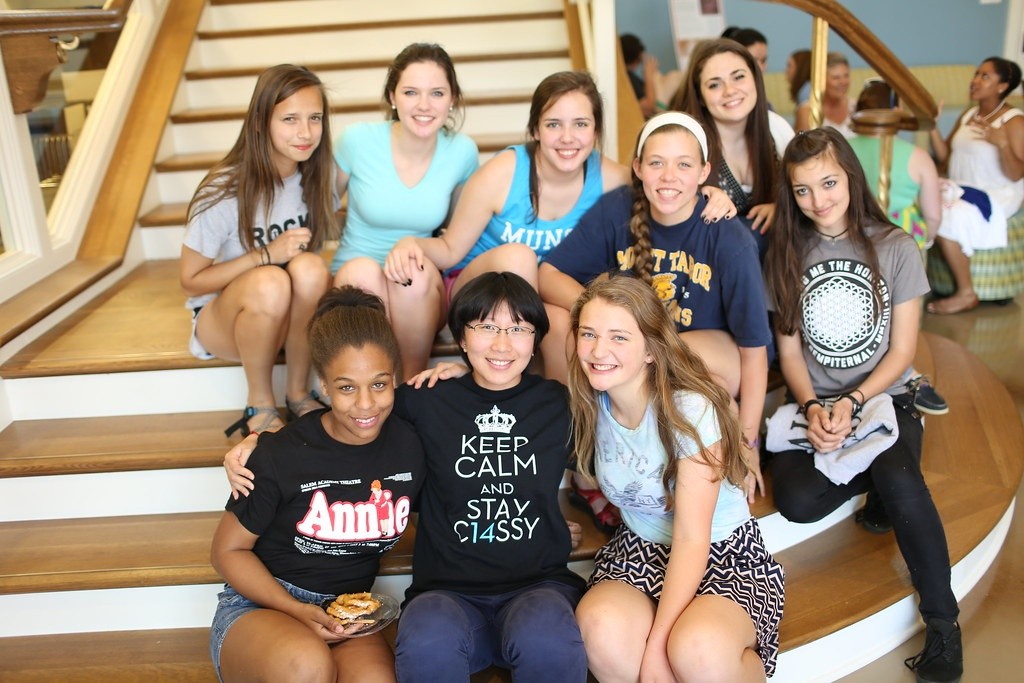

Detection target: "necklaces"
[814, 228, 849, 245]
[974, 100, 1005, 121]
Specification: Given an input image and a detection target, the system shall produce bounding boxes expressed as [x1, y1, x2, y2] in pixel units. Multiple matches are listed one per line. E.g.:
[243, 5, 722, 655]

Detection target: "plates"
[323, 592, 399, 638]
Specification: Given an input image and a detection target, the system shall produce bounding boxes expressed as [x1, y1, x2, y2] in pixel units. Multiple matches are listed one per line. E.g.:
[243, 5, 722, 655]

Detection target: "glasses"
[466, 324, 536, 339]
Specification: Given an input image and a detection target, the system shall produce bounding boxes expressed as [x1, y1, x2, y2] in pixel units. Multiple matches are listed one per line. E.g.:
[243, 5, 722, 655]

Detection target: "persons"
[770, 127, 964, 683]
[180, 63, 347, 437]
[382, 71, 737, 385]
[538, 112, 772, 529]
[223, 271, 598, 683]
[925, 57, 1024, 316]
[621, 33, 659, 110]
[668, 38, 950, 416]
[407, 276, 786, 683]
[331, 43, 480, 385]
[721, 27, 941, 247]
[208, 285, 582, 683]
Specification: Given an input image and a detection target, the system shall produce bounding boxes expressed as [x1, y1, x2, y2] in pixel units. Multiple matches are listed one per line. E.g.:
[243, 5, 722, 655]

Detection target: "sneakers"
[906, 378, 948, 414]
[904, 617, 964, 683]
[854, 492, 895, 532]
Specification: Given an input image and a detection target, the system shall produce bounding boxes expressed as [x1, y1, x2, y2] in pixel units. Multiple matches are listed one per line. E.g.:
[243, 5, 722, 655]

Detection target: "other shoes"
[928, 297, 978, 315]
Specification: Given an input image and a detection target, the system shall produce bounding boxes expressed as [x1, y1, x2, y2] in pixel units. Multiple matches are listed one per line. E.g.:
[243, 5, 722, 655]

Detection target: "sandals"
[571, 475, 624, 536]
[286, 390, 328, 422]
[225, 406, 284, 438]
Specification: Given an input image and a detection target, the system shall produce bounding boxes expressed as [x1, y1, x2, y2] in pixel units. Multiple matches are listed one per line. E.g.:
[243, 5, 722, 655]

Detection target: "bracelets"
[749, 438, 758, 446]
[802, 399, 822, 420]
[250, 431, 258, 436]
[837, 395, 860, 415]
[264, 246, 270, 265]
[1000, 142, 1009, 148]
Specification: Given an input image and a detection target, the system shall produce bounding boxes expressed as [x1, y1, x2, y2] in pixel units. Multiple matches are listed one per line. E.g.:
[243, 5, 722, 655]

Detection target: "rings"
[299, 245, 304, 249]
[981, 127, 989, 134]
